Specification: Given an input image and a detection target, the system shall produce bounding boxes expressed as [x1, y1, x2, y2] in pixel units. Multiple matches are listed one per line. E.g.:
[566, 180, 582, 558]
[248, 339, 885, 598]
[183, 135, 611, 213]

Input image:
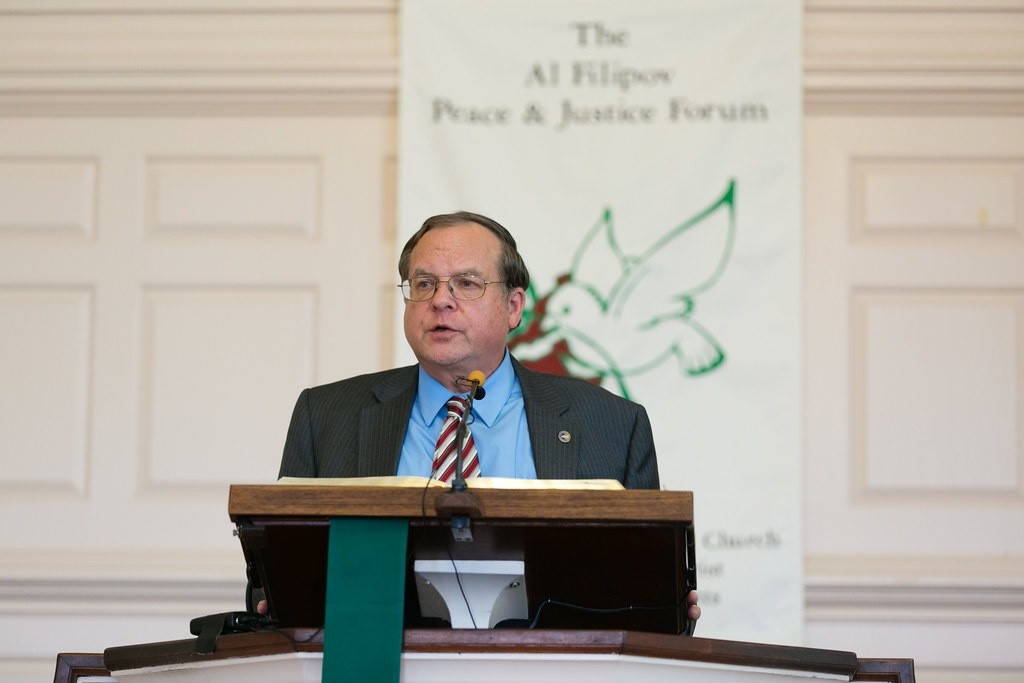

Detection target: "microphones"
[451, 370, 486, 490]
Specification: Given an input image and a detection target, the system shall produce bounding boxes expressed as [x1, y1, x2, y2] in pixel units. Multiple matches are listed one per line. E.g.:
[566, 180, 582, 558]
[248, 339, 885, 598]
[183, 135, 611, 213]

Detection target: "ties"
[431, 397, 482, 480]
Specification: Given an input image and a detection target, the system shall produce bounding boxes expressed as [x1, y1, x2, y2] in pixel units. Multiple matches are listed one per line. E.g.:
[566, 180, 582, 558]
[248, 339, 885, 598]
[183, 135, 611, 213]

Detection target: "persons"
[245, 209, 703, 621]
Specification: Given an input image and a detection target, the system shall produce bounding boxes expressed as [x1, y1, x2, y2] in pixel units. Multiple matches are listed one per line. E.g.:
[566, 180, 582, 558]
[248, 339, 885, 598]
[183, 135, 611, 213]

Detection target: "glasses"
[398, 273, 512, 302]
